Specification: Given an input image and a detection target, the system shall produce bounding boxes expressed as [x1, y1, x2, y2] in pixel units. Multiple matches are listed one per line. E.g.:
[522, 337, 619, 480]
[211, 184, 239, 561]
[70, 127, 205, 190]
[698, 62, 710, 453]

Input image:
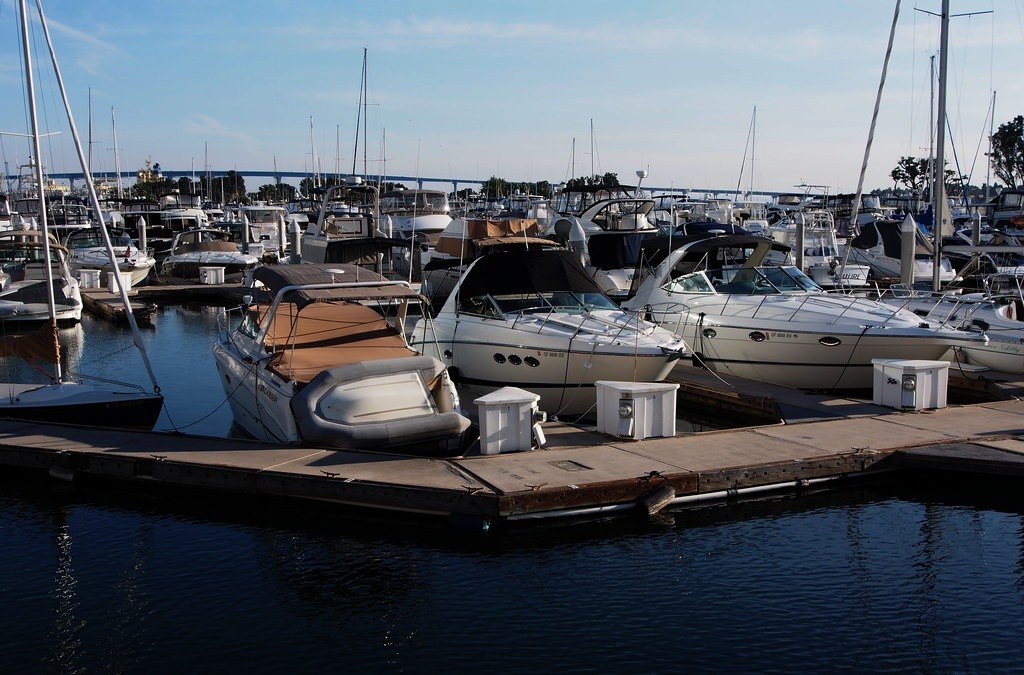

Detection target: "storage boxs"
[873, 358, 951, 411]
[108, 272, 133, 293]
[75, 269, 102, 289]
[594, 379, 681, 439]
[199, 269, 226, 284]
[472, 385, 541, 456]
[241, 269, 265, 288]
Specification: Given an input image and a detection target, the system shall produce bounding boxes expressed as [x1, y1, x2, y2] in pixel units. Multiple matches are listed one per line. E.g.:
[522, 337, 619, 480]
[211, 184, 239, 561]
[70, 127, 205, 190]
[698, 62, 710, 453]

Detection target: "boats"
[212, 259, 473, 447]
[610, 232, 987, 395]
[401, 220, 684, 416]
[879, 284, 1022, 388]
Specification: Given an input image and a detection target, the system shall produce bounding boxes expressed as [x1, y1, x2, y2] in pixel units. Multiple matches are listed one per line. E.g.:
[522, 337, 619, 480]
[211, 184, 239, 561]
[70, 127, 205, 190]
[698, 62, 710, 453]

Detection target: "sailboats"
[1, 0, 166, 433]
[1, 47, 1023, 319]
